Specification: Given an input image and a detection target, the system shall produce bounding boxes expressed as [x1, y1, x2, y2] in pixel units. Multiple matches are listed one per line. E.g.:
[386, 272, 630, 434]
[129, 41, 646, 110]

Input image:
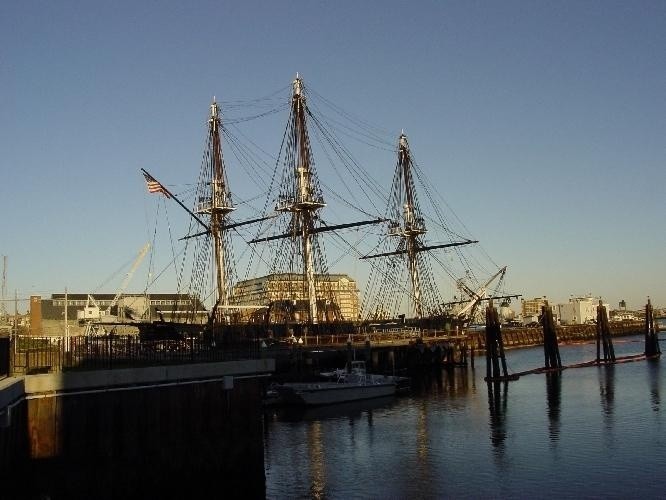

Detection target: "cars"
[525, 321, 540, 328]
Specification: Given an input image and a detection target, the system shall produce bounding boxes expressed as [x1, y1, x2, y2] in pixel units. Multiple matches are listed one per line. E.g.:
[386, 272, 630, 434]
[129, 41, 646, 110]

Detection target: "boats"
[272, 361, 396, 404]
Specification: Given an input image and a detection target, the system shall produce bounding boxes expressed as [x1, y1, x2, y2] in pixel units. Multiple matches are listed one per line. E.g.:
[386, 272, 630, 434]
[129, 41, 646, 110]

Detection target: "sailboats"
[84, 72, 523, 398]
